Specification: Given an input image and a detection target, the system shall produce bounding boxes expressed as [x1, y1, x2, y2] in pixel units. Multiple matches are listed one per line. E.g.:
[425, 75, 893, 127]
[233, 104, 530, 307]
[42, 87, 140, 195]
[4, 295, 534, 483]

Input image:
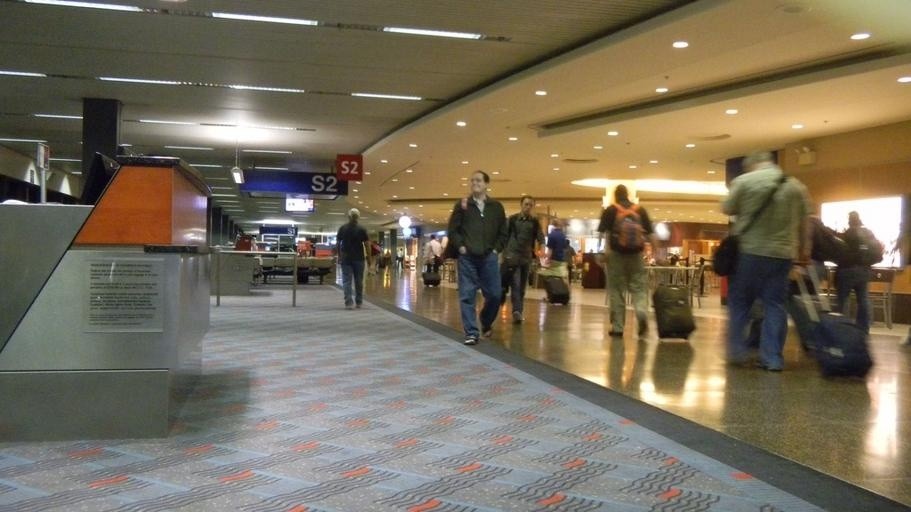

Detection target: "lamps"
[230, 143, 245, 184]
[794, 145, 816, 165]
[399, 215, 412, 228]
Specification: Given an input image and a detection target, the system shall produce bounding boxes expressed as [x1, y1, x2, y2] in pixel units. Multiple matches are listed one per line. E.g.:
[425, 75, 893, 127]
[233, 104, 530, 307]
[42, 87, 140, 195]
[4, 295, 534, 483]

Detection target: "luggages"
[420, 271, 441, 287]
[542, 275, 570, 305]
[807, 310, 873, 381]
[653, 282, 696, 341]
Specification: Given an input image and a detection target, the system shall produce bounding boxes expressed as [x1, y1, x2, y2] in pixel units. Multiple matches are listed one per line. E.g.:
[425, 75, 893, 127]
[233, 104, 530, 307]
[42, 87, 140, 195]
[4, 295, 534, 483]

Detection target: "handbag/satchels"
[434, 255, 443, 266]
[500, 259, 510, 306]
[711, 235, 742, 276]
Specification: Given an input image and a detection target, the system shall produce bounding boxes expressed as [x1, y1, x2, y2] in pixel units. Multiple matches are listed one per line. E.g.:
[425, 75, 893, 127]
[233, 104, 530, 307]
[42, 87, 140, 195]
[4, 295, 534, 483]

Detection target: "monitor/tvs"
[285, 196, 315, 214]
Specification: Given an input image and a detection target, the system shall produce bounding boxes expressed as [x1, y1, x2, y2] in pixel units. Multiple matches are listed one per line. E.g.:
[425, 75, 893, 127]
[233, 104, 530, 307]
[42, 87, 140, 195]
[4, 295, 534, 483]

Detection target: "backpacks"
[857, 227, 884, 265]
[611, 202, 646, 256]
[813, 216, 860, 270]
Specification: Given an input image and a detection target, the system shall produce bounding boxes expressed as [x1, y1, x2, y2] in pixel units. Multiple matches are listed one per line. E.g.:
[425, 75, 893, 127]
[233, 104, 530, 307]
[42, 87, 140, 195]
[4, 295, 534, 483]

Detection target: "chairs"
[263, 255, 336, 286]
[603, 255, 715, 309]
[821, 266, 896, 329]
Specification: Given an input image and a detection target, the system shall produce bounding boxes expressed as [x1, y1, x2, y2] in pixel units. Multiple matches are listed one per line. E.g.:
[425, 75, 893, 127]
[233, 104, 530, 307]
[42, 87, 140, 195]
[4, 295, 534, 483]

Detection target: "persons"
[567, 239, 577, 285]
[595, 183, 662, 339]
[713, 149, 814, 371]
[833, 210, 886, 337]
[337, 208, 372, 310]
[447, 169, 509, 345]
[543, 219, 569, 302]
[426, 233, 443, 272]
[500, 196, 547, 321]
[398, 247, 404, 269]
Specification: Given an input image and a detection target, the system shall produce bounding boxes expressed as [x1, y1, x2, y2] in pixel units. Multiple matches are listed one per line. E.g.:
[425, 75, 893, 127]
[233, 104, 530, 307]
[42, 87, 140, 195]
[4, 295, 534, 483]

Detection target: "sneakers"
[638, 321, 650, 337]
[464, 333, 480, 345]
[512, 310, 525, 323]
[480, 315, 492, 338]
[356, 302, 363, 308]
[608, 330, 624, 337]
[345, 302, 354, 309]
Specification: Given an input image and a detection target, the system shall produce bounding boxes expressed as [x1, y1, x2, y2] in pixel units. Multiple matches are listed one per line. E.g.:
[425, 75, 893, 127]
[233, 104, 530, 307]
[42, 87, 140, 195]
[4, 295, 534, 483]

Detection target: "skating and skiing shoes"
[441, 228, 463, 261]
